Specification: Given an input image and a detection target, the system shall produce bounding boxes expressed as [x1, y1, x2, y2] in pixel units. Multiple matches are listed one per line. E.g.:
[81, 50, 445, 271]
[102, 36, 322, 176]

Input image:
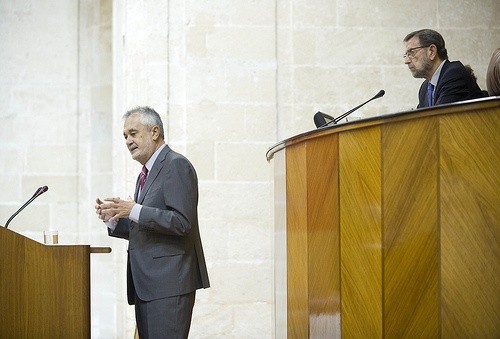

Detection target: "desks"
[266, 96, 500, 339]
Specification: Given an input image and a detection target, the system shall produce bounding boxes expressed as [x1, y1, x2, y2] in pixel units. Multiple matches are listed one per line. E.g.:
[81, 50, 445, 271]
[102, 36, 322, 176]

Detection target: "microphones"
[6, 186, 48, 228]
[318, 90, 385, 128]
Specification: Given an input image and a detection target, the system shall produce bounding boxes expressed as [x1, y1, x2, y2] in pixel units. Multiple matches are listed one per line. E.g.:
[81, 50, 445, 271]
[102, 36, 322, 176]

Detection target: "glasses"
[402, 46, 422, 57]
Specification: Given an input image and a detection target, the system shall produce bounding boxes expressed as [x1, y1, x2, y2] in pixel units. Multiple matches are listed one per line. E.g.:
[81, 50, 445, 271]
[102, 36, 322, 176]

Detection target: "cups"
[44, 230, 58, 245]
[346, 117, 361, 122]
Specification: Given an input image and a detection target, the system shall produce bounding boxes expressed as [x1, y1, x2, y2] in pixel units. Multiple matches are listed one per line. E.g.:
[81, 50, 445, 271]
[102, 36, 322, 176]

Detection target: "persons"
[403, 29, 482, 108]
[96, 106, 209, 339]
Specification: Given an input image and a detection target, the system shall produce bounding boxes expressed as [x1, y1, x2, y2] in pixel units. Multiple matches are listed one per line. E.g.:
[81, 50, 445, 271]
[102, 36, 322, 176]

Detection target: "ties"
[138, 165, 149, 191]
[427, 83, 434, 107]
